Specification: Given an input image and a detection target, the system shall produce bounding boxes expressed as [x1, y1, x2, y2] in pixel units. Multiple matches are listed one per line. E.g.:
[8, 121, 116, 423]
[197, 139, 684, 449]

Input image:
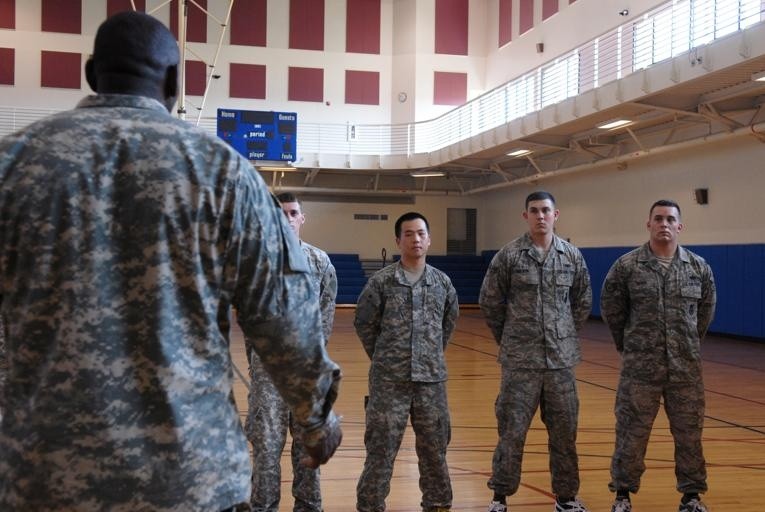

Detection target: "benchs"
[393, 256, 507, 309]
[328, 253, 368, 308]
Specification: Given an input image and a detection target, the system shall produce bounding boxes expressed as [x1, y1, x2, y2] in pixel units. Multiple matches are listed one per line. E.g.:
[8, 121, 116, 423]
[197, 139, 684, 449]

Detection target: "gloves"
[299, 420, 342, 468]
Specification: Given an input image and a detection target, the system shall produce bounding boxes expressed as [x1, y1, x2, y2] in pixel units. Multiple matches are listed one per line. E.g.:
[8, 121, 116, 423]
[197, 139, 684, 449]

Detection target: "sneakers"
[610, 496, 632, 512]
[554, 496, 589, 512]
[676, 498, 710, 512]
[489, 497, 507, 512]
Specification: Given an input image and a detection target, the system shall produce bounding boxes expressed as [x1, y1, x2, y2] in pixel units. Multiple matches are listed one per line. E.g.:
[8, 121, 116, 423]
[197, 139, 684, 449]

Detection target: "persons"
[0, 12, 342, 511]
[477, 192, 591, 512]
[243, 193, 338, 512]
[602, 200, 717, 510]
[353, 212, 460, 511]
